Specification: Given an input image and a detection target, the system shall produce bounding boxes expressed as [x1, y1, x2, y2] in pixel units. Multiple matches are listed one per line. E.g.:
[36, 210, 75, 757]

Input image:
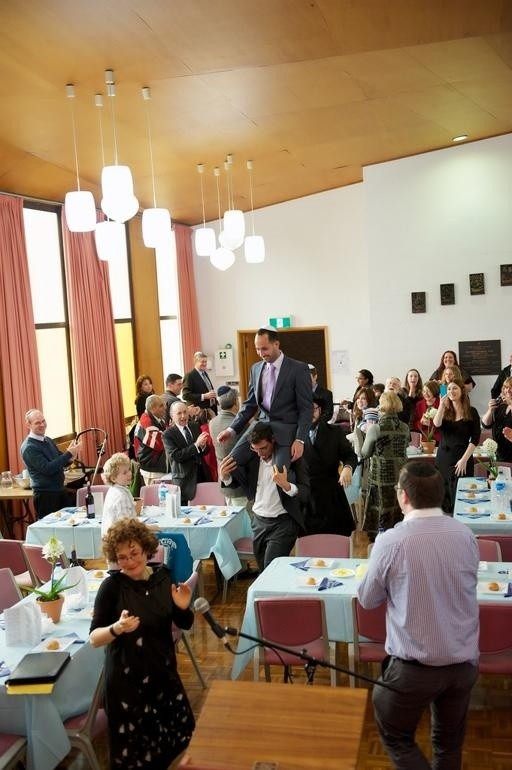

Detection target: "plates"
[457, 483, 511, 522]
[87, 569, 109, 580]
[75, 608, 94, 620]
[174, 505, 232, 525]
[299, 557, 354, 586]
[32, 633, 76, 652]
[476, 580, 509, 595]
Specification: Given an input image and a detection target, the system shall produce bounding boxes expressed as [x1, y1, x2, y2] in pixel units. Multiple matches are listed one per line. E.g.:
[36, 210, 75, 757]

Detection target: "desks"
[177, 678, 371, 769]
[231, 556, 510, 682]
[3, 571, 114, 768]
[25, 503, 256, 602]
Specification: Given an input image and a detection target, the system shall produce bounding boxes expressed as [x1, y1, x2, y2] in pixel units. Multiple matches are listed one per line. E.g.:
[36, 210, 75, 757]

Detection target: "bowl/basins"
[12, 479, 31, 489]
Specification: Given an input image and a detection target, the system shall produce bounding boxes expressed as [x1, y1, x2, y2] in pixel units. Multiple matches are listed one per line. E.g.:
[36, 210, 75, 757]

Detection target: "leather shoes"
[238, 567, 260, 580]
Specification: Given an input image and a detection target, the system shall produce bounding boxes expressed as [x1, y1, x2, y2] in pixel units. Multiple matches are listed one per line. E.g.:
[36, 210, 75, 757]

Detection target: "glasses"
[115, 549, 144, 564]
[250, 444, 268, 451]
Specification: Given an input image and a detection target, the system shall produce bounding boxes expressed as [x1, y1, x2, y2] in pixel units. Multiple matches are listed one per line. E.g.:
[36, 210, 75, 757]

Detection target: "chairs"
[2, 732, 29, 769]
[21, 544, 54, 586]
[58, 657, 111, 768]
[473, 600, 510, 675]
[254, 595, 340, 686]
[2, 405, 511, 561]
[1, 567, 23, 614]
[347, 595, 392, 690]
[170, 572, 207, 689]
[2, 539, 40, 598]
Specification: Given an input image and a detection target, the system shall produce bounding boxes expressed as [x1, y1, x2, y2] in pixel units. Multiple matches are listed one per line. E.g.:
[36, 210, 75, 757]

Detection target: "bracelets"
[107, 622, 120, 638]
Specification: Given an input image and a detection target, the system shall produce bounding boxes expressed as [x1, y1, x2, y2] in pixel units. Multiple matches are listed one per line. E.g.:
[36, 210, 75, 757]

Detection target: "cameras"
[496, 396, 502, 404]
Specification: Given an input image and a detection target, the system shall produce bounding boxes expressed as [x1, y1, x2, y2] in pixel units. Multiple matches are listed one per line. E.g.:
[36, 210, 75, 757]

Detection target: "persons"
[97, 452, 138, 568]
[19, 409, 82, 518]
[307, 349, 512, 537]
[87, 518, 193, 769]
[219, 423, 310, 574]
[355, 462, 480, 770]
[217, 325, 313, 503]
[209, 386, 260, 582]
[125, 350, 216, 505]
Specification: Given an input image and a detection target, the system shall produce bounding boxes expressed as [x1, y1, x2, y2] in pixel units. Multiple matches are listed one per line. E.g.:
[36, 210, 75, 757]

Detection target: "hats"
[217, 386, 230, 396]
[308, 364, 315, 369]
[312, 392, 327, 409]
[260, 325, 278, 332]
[250, 421, 270, 432]
[406, 461, 436, 477]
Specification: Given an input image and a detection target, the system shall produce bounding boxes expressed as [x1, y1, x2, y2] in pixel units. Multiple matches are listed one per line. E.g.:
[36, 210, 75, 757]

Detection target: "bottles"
[70, 543, 80, 566]
[158, 480, 168, 513]
[52, 562, 65, 588]
[494, 471, 508, 511]
[85, 481, 95, 518]
[209, 389, 215, 405]
[376, 527, 384, 541]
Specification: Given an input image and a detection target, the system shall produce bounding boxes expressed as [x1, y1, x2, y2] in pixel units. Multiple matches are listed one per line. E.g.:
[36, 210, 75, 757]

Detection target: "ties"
[184, 425, 201, 465]
[309, 430, 317, 444]
[262, 364, 276, 411]
[202, 373, 214, 405]
[160, 420, 166, 429]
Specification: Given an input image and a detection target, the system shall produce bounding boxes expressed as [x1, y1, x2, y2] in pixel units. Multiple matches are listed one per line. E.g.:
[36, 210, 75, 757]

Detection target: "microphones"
[194, 597, 233, 651]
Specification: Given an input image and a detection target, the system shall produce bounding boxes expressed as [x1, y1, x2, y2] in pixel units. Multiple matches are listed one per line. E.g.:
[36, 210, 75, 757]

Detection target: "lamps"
[91, 93, 126, 263]
[194, 163, 217, 256]
[99, 69, 141, 223]
[244, 159, 265, 265]
[139, 86, 173, 249]
[218, 160, 243, 251]
[210, 167, 236, 270]
[64, 83, 97, 234]
[224, 154, 246, 240]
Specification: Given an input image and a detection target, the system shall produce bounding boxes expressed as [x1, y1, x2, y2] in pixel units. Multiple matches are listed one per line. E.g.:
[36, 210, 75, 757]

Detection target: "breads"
[489, 582, 499, 591]
[201, 506, 206, 510]
[47, 640, 59, 650]
[470, 507, 477, 512]
[499, 512, 506, 520]
[468, 492, 475, 498]
[307, 577, 315, 585]
[183, 518, 190, 523]
[470, 483, 477, 489]
[95, 571, 104, 578]
[316, 559, 324, 566]
[220, 511, 226, 516]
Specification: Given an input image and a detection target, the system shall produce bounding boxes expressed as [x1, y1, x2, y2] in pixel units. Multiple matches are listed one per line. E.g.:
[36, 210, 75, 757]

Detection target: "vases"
[35, 595, 65, 624]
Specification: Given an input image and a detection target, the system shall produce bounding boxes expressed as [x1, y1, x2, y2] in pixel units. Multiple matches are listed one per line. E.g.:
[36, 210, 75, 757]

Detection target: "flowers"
[20, 536, 82, 600]
